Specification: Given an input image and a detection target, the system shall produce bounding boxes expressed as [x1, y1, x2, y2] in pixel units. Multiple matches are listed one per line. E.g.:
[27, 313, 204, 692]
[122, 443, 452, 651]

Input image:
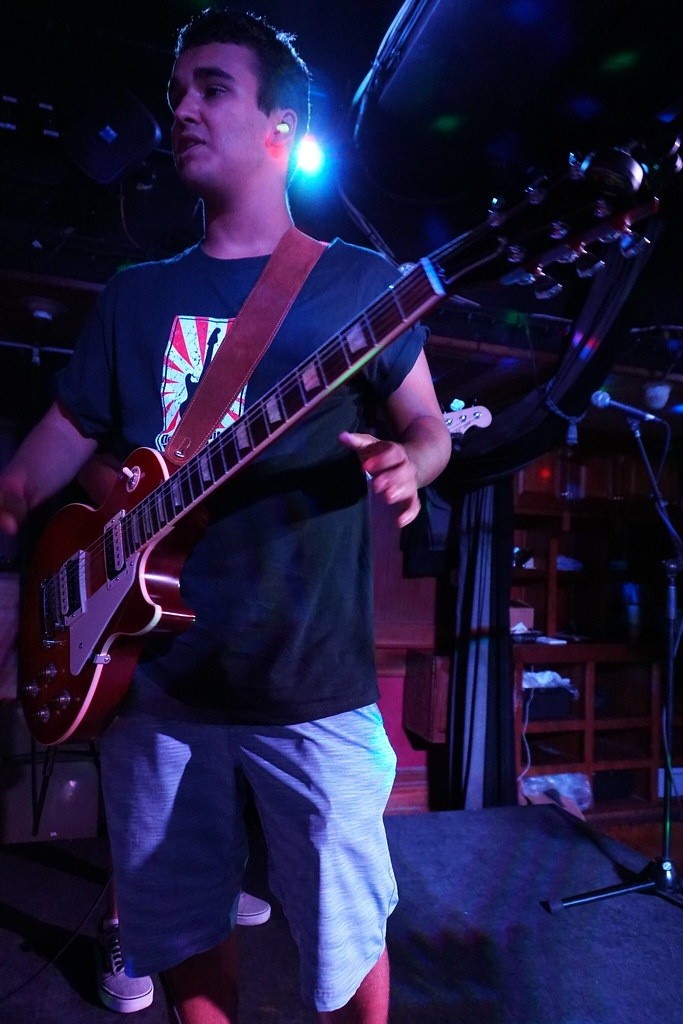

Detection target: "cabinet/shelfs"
[512, 448, 683, 828]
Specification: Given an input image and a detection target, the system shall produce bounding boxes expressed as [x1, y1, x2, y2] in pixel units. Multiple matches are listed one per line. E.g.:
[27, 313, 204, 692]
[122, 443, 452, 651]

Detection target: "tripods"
[544, 413, 683, 912]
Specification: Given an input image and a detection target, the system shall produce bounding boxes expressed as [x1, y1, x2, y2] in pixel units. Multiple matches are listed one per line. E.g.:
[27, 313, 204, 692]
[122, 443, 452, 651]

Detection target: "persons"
[0, 5, 451, 1024]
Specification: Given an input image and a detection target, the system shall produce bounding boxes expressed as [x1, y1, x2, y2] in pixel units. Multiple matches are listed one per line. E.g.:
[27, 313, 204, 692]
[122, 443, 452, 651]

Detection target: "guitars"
[20, 135, 683, 748]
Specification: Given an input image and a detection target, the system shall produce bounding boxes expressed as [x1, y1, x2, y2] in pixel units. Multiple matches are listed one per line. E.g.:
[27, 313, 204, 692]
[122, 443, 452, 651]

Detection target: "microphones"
[591, 389, 661, 422]
